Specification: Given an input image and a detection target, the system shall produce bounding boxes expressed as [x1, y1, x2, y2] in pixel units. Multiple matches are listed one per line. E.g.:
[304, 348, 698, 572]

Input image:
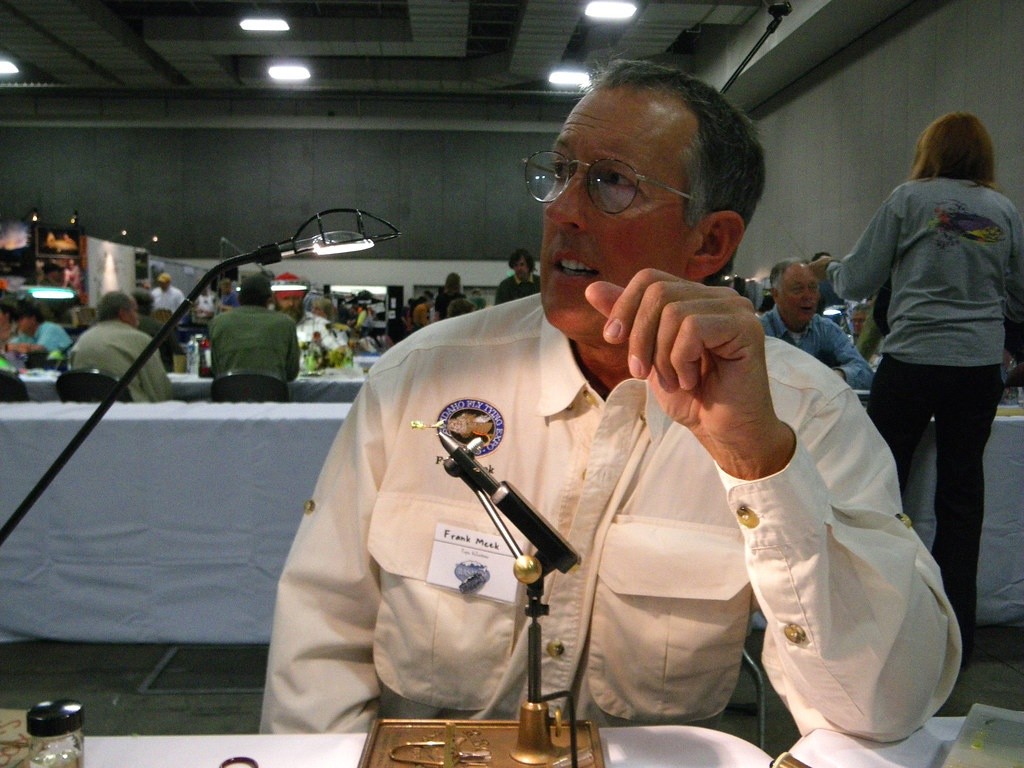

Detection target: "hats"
[158, 272, 172, 282]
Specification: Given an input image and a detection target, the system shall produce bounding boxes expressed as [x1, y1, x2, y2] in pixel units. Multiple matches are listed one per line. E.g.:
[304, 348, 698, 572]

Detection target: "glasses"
[523, 150, 694, 214]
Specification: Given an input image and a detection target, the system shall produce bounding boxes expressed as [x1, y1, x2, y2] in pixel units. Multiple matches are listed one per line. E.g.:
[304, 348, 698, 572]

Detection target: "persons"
[0, 292, 76, 375]
[197, 275, 241, 318]
[385, 272, 486, 347]
[758, 251, 875, 402]
[261, 59, 964, 744]
[267, 272, 368, 370]
[207, 275, 301, 405]
[150, 273, 191, 374]
[65, 289, 175, 403]
[63, 260, 83, 296]
[130, 289, 186, 372]
[496, 247, 541, 307]
[806, 112, 1024, 671]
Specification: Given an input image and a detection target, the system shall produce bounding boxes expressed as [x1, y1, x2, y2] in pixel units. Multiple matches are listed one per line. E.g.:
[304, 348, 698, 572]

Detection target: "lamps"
[0, 205, 402, 535]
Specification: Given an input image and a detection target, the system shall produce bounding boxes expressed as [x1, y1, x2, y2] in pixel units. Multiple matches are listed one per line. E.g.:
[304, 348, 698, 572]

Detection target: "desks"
[0, 356, 380, 644]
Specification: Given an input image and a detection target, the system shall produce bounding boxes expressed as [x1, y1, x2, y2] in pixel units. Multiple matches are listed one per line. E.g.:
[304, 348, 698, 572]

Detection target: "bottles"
[195, 334, 210, 377]
[186, 335, 199, 376]
[25, 699, 84, 768]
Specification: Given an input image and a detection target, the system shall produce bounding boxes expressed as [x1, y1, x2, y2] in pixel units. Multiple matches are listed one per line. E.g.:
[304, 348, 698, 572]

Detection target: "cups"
[173, 352, 186, 373]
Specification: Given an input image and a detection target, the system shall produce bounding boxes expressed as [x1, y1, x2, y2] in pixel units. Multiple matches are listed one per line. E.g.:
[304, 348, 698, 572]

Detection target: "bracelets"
[824, 260, 841, 270]
